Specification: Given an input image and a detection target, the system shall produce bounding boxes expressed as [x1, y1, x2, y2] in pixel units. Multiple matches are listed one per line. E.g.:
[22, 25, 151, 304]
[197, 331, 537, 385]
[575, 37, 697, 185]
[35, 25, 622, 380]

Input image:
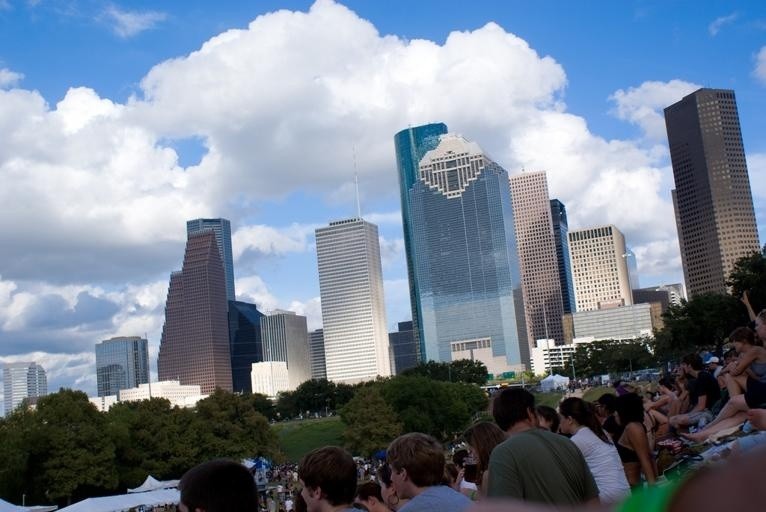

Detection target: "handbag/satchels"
[656, 438, 705, 476]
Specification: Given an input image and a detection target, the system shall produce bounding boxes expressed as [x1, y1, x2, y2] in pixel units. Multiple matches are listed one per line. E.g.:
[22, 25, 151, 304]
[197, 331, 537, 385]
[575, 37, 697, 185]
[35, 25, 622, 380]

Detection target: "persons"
[178, 288, 765, 512]
